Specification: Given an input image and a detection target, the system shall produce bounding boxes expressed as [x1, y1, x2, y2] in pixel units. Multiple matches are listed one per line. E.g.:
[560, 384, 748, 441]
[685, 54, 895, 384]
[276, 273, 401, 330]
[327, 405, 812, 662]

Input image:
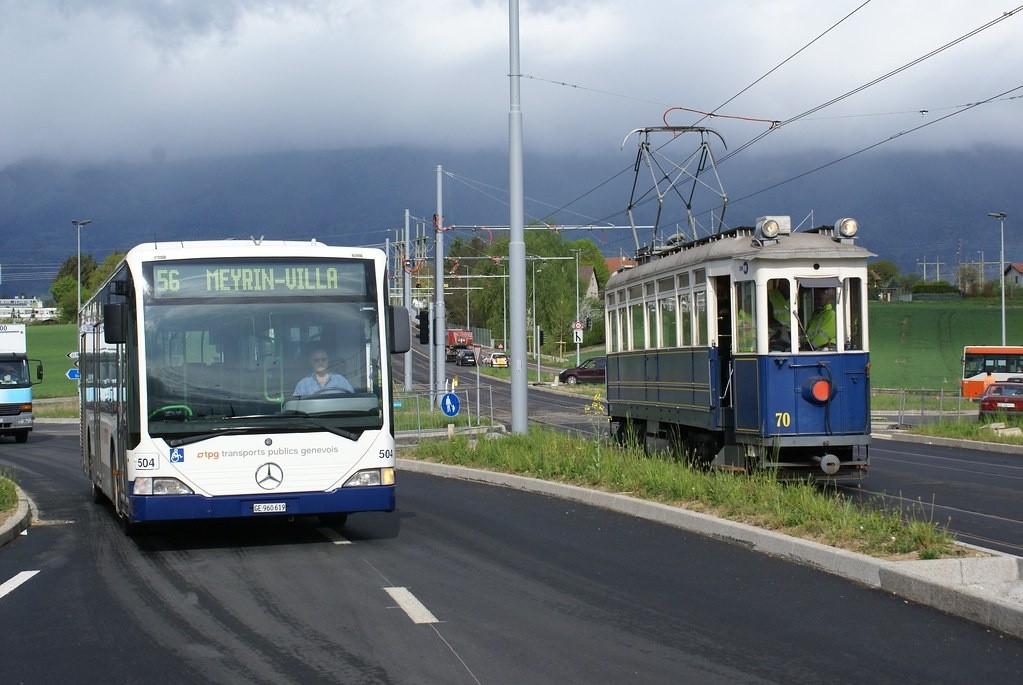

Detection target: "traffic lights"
[415, 310, 430, 345]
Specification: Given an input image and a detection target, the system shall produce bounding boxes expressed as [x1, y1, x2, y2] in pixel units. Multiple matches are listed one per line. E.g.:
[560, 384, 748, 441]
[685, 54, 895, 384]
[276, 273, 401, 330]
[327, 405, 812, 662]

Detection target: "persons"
[767, 279, 791, 353]
[293, 349, 355, 396]
[738, 295, 775, 351]
[803, 292, 837, 352]
[984, 371, 995, 395]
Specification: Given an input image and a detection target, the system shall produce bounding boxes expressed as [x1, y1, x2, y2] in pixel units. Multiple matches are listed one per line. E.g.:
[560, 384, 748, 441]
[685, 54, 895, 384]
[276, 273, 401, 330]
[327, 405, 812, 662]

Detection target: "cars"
[977, 381, 1023, 422]
[456, 350, 476, 366]
[486, 352, 509, 368]
[559, 355, 606, 386]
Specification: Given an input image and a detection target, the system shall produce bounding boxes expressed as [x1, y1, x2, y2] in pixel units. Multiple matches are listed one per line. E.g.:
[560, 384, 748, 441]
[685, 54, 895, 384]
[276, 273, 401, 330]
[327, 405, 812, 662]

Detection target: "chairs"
[147, 361, 293, 421]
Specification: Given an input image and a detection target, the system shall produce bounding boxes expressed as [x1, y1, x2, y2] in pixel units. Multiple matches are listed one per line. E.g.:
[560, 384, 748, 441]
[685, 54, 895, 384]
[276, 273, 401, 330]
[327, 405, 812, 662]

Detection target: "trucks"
[0, 323, 43, 444]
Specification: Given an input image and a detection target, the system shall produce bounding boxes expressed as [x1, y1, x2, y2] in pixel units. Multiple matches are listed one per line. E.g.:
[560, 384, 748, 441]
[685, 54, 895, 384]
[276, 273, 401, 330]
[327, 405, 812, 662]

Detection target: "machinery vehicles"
[445, 328, 482, 362]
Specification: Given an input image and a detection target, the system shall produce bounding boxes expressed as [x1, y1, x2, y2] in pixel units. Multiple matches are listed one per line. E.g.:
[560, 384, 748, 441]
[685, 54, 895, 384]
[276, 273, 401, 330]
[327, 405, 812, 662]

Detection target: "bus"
[0, 308, 66, 324]
[77, 236, 413, 540]
[604, 215, 882, 490]
[961, 345, 1023, 400]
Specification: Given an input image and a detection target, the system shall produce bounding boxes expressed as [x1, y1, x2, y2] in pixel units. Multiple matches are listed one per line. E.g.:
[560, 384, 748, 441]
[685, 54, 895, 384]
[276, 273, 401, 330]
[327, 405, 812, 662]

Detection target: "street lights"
[570, 249, 580, 366]
[987, 209, 1008, 347]
[71, 214, 92, 314]
